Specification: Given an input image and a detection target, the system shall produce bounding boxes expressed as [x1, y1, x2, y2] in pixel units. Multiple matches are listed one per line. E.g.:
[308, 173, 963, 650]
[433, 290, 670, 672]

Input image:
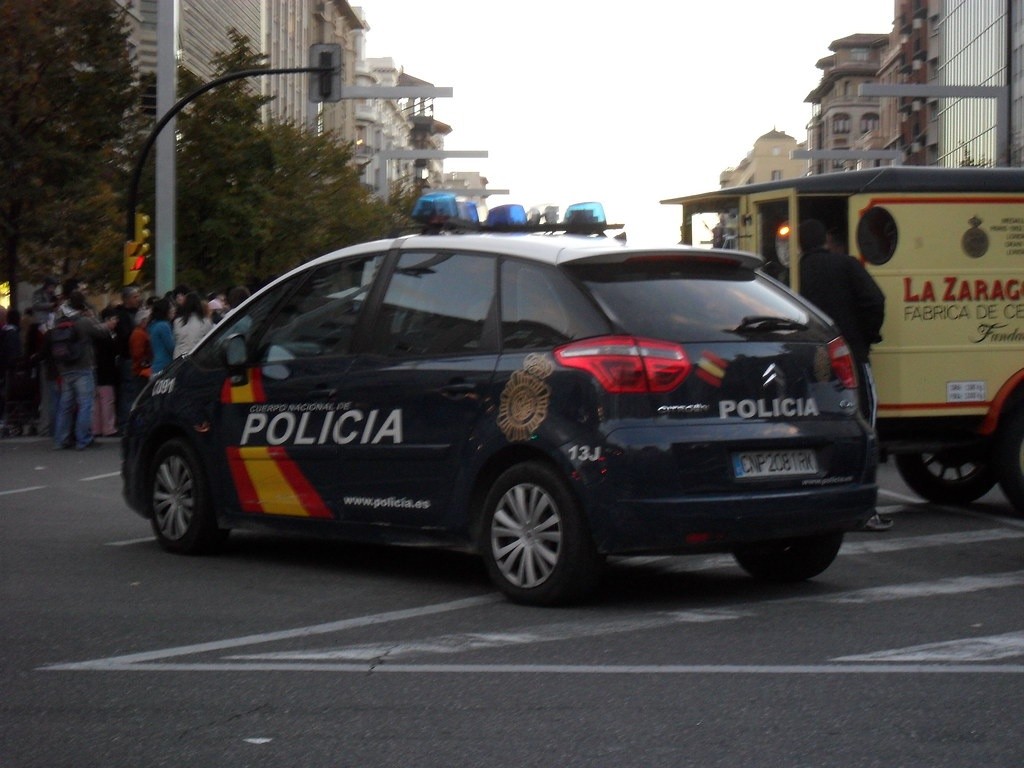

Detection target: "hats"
[135, 308, 152, 320]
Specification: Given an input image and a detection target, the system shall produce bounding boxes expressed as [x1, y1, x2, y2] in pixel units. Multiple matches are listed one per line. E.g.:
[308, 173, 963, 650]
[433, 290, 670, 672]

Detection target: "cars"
[126, 189, 880, 607]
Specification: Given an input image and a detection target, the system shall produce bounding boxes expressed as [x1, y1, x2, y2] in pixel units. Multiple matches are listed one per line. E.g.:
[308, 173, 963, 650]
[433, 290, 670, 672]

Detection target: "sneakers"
[863, 513, 893, 530]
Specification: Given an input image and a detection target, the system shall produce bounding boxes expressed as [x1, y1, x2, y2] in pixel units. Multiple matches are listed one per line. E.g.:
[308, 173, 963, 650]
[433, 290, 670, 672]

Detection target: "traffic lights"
[122, 240, 145, 287]
[127, 211, 152, 265]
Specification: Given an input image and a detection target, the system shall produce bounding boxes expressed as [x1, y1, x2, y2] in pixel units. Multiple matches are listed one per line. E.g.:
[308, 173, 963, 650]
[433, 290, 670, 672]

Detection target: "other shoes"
[76, 440, 101, 450]
[64, 439, 75, 448]
[95, 434, 102, 437]
[107, 431, 124, 437]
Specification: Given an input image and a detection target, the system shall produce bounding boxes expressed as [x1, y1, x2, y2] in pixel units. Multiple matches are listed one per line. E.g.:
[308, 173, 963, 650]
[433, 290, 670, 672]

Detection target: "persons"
[0, 274, 253, 449]
[784, 218, 895, 530]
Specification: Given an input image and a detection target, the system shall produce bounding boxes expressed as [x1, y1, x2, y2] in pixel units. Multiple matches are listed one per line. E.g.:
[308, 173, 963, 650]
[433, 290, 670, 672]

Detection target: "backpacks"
[48, 309, 86, 363]
